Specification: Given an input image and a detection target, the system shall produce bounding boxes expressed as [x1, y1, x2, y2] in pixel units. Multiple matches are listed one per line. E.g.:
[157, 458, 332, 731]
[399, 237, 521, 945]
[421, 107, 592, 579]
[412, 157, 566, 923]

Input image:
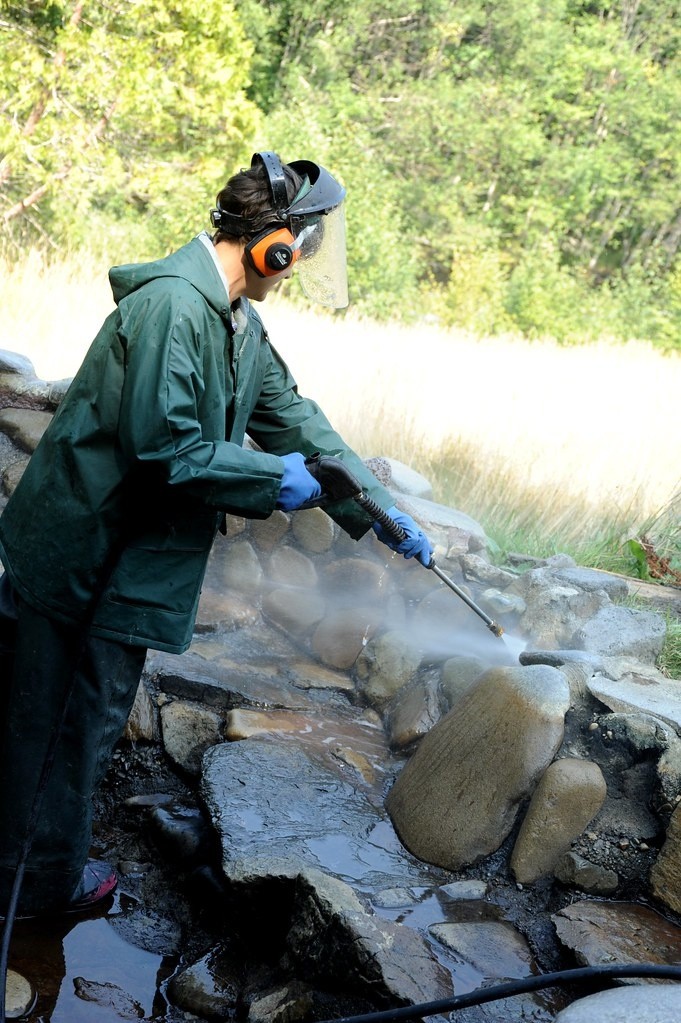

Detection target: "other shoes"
[0, 861, 119, 920]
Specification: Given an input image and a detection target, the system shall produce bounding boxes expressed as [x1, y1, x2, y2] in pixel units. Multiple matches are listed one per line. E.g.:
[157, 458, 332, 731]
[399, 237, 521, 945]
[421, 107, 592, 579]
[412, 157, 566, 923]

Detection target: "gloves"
[372, 505, 434, 565]
[276, 452, 322, 513]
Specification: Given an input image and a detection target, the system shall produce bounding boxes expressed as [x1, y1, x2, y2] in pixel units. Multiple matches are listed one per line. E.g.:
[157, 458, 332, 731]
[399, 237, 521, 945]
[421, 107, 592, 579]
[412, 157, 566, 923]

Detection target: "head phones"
[245, 223, 324, 278]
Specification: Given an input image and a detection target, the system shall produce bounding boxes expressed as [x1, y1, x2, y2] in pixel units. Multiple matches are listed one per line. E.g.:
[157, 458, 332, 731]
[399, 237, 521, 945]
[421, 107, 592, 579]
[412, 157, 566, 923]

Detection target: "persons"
[0, 155, 434, 918]
